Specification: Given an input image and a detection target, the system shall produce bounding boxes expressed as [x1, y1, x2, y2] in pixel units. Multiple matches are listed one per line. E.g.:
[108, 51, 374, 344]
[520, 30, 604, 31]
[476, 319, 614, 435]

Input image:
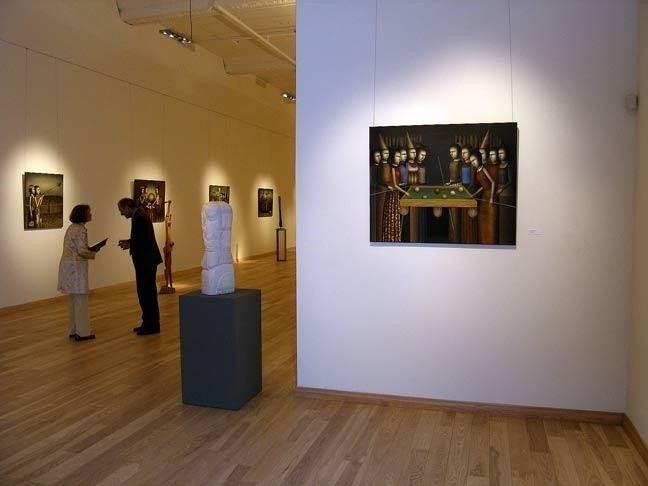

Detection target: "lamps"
[159, 0, 195, 46]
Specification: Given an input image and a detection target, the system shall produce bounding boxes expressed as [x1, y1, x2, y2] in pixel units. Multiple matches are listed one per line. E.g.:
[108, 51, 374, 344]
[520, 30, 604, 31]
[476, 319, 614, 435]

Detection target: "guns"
[37, 183, 60, 200]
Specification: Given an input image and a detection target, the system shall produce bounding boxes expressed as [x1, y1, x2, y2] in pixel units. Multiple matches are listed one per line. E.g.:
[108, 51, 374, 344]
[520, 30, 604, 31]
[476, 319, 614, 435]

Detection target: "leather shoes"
[69, 333, 95, 341]
[133, 327, 160, 335]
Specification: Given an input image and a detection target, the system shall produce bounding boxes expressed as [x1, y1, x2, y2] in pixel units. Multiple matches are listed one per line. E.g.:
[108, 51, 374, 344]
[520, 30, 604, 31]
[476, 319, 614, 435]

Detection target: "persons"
[116, 198, 163, 335]
[27, 185, 44, 229]
[57, 204, 101, 340]
[136, 186, 163, 214]
[370, 143, 516, 245]
[211, 186, 227, 202]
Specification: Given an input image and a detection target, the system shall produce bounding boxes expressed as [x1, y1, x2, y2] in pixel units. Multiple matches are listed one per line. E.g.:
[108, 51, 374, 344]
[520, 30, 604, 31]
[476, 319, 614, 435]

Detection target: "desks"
[397, 185, 479, 241]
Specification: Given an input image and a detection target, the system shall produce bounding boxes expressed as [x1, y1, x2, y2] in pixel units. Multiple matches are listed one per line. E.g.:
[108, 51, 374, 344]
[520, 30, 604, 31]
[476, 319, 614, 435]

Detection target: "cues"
[436, 155, 455, 231]
[370, 187, 405, 196]
[456, 195, 516, 209]
